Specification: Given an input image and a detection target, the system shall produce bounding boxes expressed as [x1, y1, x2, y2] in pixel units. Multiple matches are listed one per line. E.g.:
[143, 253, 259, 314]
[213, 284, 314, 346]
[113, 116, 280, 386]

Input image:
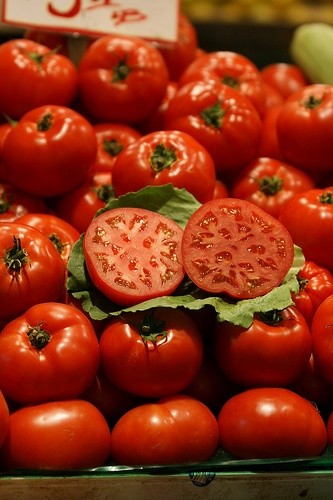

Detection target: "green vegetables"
[65, 182, 307, 329]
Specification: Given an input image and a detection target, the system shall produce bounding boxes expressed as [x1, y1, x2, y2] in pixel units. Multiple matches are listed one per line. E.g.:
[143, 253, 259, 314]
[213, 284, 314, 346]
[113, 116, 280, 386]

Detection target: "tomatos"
[0, 15, 333, 468]
